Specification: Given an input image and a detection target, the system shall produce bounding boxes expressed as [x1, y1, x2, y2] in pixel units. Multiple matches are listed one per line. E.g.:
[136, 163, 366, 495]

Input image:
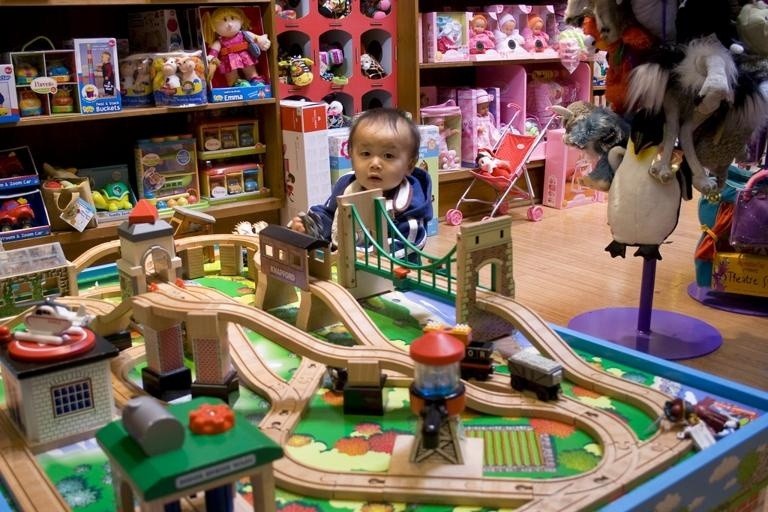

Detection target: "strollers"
[446, 102, 558, 225]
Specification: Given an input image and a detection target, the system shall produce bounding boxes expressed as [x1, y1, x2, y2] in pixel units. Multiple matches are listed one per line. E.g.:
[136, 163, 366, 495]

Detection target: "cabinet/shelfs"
[0, 1, 273, 265]
[258, 1, 398, 251]
[405, 2, 610, 219]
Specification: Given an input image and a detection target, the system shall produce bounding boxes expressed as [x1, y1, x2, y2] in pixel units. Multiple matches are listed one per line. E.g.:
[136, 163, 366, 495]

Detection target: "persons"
[421, 3, 597, 63]
[419, 85, 501, 167]
[473, 147, 514, 182]
[290, 108, 433, 265]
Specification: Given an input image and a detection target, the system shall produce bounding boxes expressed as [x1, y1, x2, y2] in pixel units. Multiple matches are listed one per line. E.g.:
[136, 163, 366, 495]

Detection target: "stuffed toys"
[604, 107, 694, 262]
[548, 100, 630, 194]
[275, 0, 397, 127]
